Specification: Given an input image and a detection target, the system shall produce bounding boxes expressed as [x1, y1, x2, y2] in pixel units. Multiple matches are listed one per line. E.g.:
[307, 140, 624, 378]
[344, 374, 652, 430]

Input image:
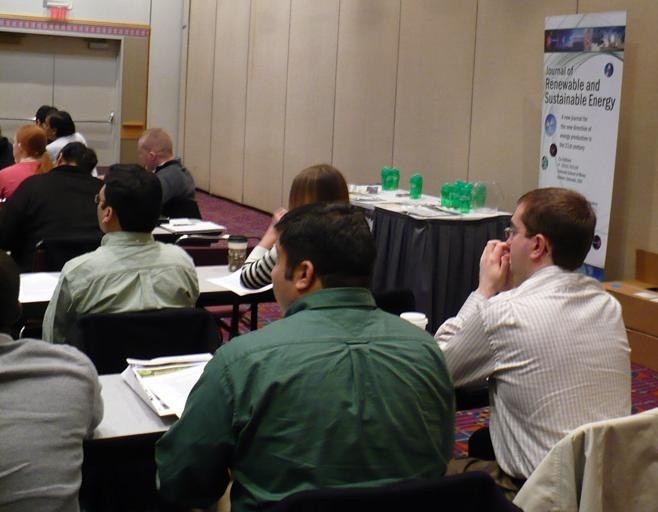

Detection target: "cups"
[398, 310, 429, 331]
[227, 235, 249, 273]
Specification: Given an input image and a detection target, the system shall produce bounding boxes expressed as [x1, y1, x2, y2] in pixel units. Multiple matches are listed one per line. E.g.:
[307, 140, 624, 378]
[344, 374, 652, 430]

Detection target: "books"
[345, 180, 383, 198]
[122, 344, 227, 425]
[374, 200, 452, 220]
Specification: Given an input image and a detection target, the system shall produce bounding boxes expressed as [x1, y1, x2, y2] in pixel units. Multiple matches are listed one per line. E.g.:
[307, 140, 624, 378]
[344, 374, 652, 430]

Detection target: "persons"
[0, 252, 107, 510]
[153, 199, 458, 512]
[237, 164, 350, 291]
[429, 187, 634, 492]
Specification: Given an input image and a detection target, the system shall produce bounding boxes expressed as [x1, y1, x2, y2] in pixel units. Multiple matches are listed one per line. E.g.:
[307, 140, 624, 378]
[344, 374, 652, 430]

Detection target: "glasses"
[93, 192, 113, 208]
[502, 225, 538, 240]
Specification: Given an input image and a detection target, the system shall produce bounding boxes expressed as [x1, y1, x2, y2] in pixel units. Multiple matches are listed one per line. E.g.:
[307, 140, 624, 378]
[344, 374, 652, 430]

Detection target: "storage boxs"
[600, 246, 657, 338]
[622, 328, 657, 375]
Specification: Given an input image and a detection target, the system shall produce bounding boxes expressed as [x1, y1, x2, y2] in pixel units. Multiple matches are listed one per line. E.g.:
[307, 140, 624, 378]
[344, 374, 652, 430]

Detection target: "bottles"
[381, 167, 487, 213]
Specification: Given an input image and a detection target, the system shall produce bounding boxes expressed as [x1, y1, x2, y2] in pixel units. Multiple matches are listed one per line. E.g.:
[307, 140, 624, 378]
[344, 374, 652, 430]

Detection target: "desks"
[342, 180, 516, 404]
[146, 213, 229, 241]
[14, 260, 282, 350]
[73, 356, 212, 443]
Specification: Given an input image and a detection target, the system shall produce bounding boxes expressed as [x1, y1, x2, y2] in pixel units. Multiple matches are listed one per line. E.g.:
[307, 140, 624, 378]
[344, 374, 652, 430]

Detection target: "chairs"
[65, 306, 222, 376]
[177, 229, 264, 343]
[510, 406, 656, 512]
[261, 472, 511, 512]
